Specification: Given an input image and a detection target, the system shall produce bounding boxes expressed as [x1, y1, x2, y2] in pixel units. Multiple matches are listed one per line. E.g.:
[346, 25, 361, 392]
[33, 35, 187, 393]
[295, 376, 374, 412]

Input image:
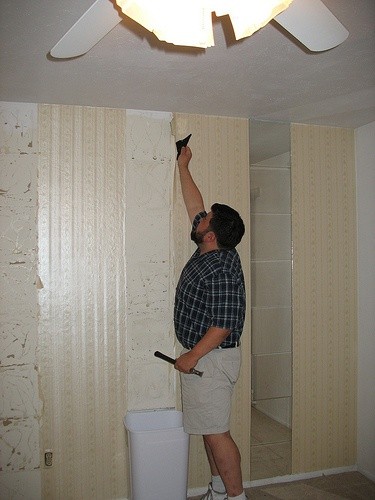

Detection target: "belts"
[185, 342, 240, 350]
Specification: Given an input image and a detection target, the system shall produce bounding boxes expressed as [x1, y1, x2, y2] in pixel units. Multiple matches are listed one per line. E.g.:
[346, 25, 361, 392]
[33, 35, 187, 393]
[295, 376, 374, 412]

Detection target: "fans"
[49, 0, 349, 58]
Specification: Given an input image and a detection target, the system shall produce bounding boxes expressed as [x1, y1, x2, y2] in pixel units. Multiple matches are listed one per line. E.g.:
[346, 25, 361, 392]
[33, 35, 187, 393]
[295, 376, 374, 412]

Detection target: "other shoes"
[199, 482, 227, 500]
[224, 496, 248, 500]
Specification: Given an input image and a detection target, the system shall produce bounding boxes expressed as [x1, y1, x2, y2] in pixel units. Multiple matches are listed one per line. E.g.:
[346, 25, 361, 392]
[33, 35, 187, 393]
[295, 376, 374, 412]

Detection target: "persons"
[174, 145, 247, 500]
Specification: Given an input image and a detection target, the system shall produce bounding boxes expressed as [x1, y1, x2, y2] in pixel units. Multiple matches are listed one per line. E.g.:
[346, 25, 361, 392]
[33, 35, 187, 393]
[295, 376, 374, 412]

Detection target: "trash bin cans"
[122, 406, 189, 500]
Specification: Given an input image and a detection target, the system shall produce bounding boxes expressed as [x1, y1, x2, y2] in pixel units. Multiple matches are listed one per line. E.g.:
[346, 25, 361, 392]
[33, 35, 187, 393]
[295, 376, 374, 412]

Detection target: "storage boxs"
[124, 411, 190, 500]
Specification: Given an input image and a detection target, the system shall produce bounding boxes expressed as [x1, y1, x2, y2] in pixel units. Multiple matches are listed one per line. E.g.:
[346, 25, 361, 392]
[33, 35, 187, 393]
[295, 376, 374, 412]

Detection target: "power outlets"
[44, 450, 53, 468]
